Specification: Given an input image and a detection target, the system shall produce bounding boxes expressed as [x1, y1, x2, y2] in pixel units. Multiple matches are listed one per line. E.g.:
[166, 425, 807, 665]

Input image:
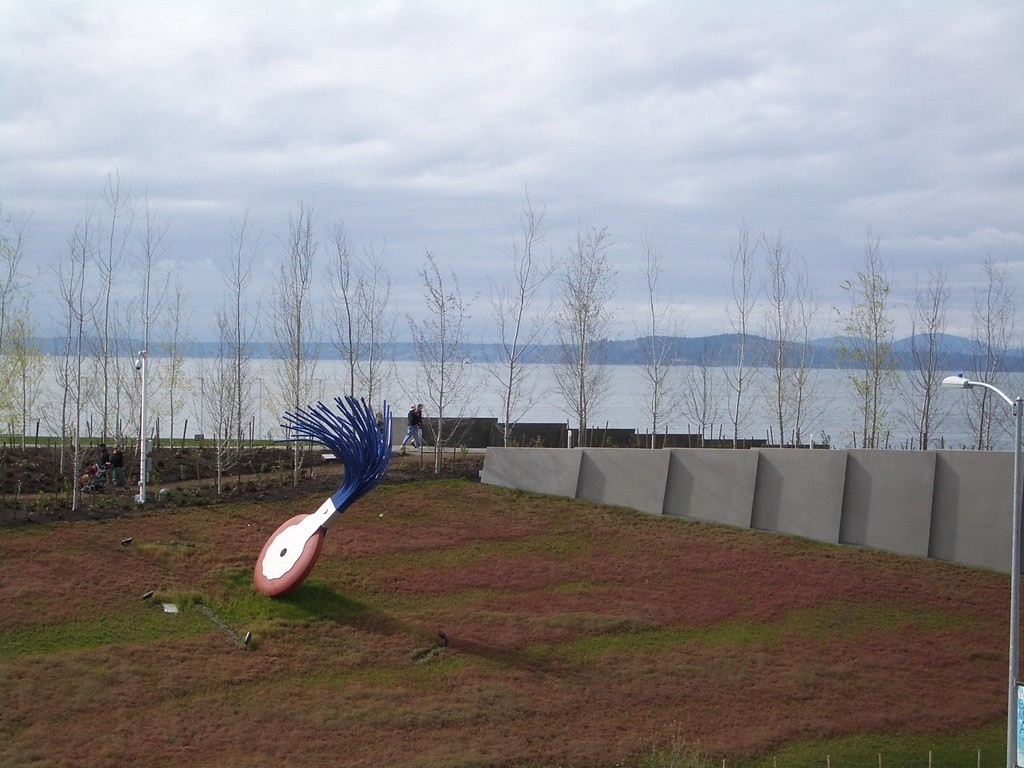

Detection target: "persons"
[401, 404, 424, 448]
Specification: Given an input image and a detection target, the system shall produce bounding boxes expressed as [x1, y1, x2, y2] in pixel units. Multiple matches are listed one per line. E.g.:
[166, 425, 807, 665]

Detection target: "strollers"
[81, 462, 116, 493]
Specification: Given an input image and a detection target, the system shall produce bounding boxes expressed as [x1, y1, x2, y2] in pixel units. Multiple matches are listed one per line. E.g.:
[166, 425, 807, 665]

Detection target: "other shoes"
[401, 445, 409, 449]
[417, 446, 425, 449]
[411, 442, 417, 448]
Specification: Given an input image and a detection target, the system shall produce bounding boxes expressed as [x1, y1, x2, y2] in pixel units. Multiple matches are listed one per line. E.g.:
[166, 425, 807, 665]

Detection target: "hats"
[410, 404, 417, 410]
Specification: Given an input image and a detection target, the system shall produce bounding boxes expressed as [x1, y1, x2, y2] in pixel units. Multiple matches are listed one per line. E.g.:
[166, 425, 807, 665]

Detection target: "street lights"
[942, 374, 1024, 768]
[134, 350, 146, 503]
